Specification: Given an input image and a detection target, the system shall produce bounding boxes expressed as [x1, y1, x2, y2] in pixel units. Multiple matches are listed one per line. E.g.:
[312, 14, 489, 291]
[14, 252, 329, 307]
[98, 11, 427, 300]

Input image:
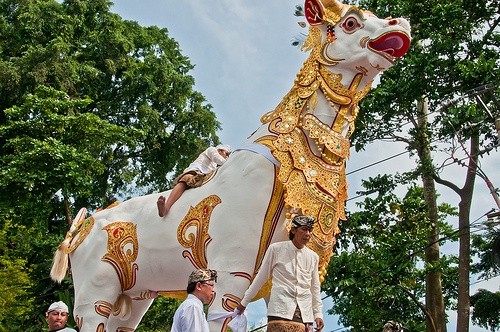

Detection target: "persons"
[157, 144, 232, 217]
[382, 321, 403, 332]
[170, 269, 218, 332]
[45, 301, 77, 332]
[237, 215, 324, 332]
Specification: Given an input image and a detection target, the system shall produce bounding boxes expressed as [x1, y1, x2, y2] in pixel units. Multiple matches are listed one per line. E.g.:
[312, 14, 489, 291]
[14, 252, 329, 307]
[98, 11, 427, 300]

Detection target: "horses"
[49, 0, 412, 332]
